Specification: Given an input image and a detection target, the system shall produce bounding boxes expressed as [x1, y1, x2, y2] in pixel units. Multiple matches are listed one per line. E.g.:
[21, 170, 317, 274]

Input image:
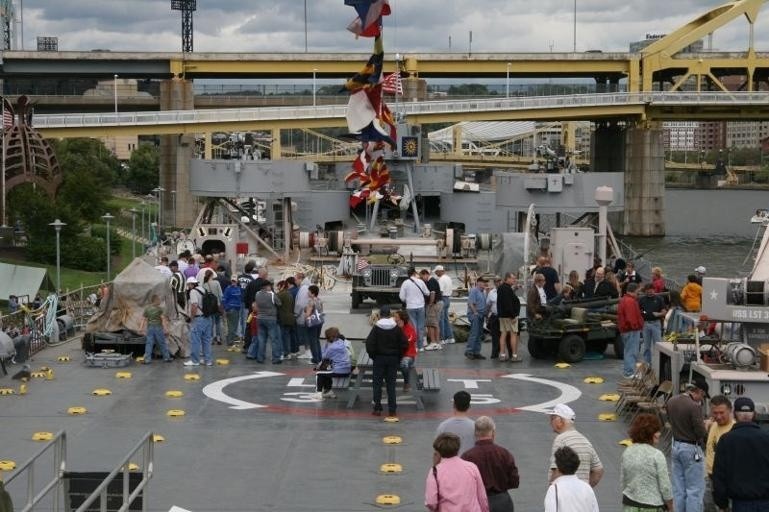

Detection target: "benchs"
[422, 368, 441, 391]
[330, 374, 351, 389]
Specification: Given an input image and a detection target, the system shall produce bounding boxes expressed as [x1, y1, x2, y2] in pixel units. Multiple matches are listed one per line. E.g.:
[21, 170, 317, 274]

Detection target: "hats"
[495, 276, 501, 282]
[688, 275, 697, 283]
[694, 266, 706, 273]
[166, 261, 178, 266]
[231, 275, 238, 282]
[187, 276, 198, 283]
[691, 379, 710, 399]
[261, 281, 274, 285]
[734, 397, 754, 412]
[544, 404, 576, 424]
[477, 277, 488, 283]
[652, 267, 662, 275]
[432, 265, 444, 273]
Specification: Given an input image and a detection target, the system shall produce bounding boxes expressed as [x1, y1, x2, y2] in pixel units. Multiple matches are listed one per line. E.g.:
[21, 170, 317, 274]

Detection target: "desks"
[346, 347, 424, 411]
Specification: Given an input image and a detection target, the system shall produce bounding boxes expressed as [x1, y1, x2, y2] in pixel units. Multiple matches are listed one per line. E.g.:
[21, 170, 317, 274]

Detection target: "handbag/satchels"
[423, 304, 441, 328]
[306, 309, 324, 327]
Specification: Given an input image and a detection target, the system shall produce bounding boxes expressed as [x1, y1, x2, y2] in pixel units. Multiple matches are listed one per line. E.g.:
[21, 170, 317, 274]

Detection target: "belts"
[195, 315, 204, 317]
[674, 439, 701, 445]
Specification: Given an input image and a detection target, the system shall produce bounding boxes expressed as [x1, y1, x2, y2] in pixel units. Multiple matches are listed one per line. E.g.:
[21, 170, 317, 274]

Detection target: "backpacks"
[193, 288, 218, 317]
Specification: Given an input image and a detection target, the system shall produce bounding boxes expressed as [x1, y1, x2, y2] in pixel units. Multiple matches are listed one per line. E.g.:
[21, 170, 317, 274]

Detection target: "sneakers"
[144, 358, 213, 366]
[372, 383, 412, 417]
[416, 336, 455, 352]
[311, 392, 323, 400]
[623, 371, 642, 380]
[322, 390, 336, 399]
[226, 345, 322, 365]
[464, 352, 523, 362]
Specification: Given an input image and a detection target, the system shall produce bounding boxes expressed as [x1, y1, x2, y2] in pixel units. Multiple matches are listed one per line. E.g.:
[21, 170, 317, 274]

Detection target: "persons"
[400, 266, 456, 352]
[0, 280, 110, 365]
[303, 285, 325, 365]
[236, 259, 315, 366]
[423, 432, 491, 512]
[139, 294, 174, 365]
[433, 390, 476, 466]
[666, 379, 711, 512]
[543, 404, 603, 487]
[711, 397, 769, 511]
[651, 268, 666, 293]
[702, 396, 737, 510]
[393, 310, 417, 391]
[241, 145, 263, 161]
[310, 326, 356, 400]
[618, 283, 644, 378]
[460, 415, 520, 512]
[639, 284, 667, 371]
[525, 257, 559, 322]
[182, 277, 213, 366]
[465, 272, 523, 361]
[153, 250, 242, 348]
[681, 266, 706, 312]
[621, 413, 675, 511]
[365, 305, 407, 415]
[544, 446, 599, 512]
[558, 255, 642, 302]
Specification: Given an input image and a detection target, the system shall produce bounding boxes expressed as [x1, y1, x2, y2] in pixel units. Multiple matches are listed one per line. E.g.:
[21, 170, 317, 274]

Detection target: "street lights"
[48, 219, 67, 297]
[394, 52, 400, 111]
[114, 74, 118, 112]
[102, 212, 115, 281]
[507, 62, 512, 97]
[128, 185, 177, 259]
[312, 68, 318, 106]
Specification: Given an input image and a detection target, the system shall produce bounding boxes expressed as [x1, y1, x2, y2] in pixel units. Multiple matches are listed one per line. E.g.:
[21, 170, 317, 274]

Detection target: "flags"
[345, 0, 404, 207]
[359, 257, 369, 271]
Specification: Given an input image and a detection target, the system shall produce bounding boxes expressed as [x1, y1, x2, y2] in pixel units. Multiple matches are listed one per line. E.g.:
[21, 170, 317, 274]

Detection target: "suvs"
[350, 247, 410, 310]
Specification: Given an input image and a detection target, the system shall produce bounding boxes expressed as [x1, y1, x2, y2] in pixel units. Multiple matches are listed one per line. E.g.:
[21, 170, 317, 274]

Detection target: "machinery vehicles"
[526, 287, 683, 365]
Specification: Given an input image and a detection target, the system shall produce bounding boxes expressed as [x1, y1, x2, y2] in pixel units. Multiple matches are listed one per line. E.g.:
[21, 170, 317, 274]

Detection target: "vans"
[428, 91, 448, 99]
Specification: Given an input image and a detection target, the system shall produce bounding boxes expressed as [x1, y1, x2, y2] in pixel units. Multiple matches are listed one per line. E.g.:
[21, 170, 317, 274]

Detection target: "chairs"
[614, 359, 674, 432]
